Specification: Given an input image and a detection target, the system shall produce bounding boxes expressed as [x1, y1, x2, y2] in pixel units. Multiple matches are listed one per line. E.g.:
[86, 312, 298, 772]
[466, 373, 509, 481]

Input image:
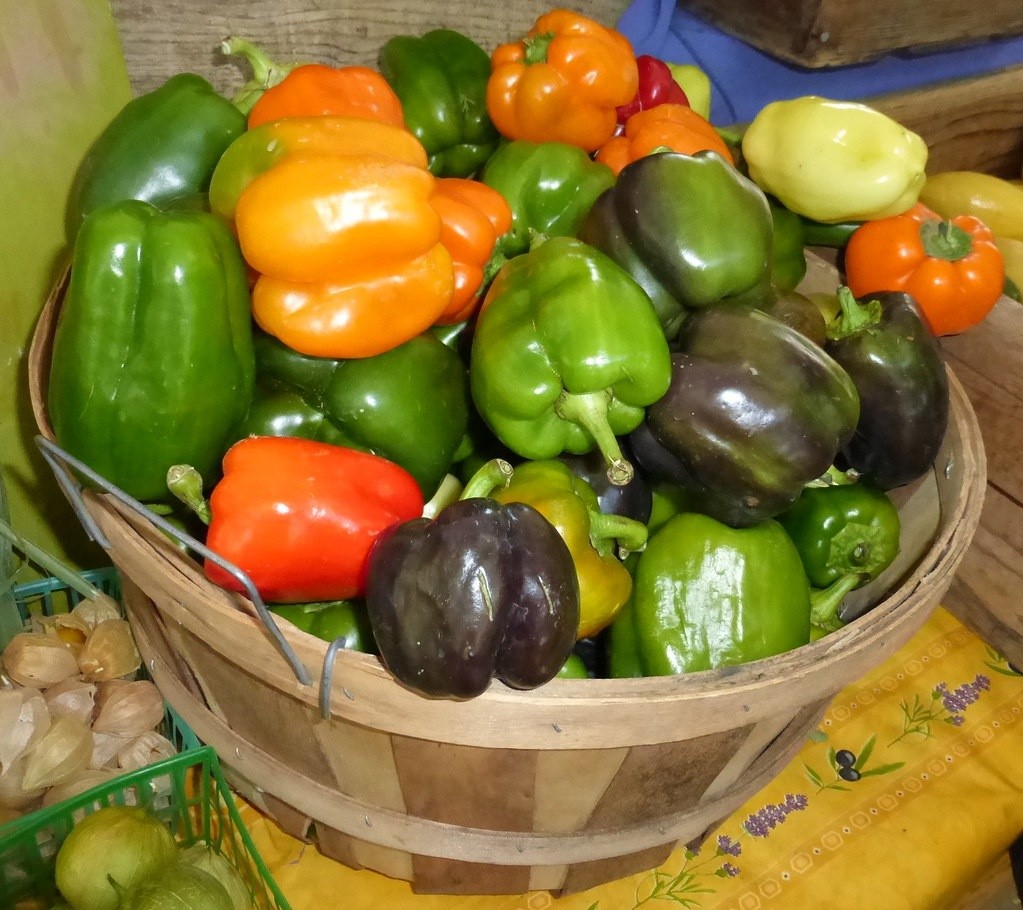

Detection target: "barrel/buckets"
[26, 243, 989, 896]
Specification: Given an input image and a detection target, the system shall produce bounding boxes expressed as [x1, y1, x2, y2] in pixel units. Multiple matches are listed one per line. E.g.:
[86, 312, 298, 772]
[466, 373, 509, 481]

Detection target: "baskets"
[0, 566, 202, 833]
[0, 747, 293, 910]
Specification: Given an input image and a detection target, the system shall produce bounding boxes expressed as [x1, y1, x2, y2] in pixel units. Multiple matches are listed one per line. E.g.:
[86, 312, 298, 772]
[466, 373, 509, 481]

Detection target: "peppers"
[44, 11, 1023, 696]
